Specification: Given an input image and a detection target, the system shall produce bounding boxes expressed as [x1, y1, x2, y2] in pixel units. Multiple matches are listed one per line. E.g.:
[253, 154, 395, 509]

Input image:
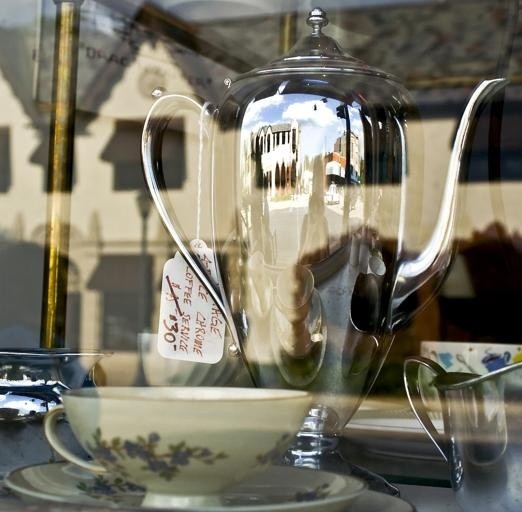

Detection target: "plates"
[340, 417, 446, 461]
[1, 461, 415, 511]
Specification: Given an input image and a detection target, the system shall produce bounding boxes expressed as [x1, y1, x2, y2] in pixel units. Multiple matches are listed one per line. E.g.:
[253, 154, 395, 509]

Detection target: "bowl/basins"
[0, 346, 108, 425]
[79, 326, 242, 386]
[419, 339, 522, 434]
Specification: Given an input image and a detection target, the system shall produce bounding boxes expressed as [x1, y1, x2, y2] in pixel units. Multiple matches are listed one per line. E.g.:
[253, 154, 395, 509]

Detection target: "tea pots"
[139, 4, 506, 492]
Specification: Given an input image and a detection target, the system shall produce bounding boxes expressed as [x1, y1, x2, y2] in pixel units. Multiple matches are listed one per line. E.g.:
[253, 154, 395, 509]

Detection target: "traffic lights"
[336, 103, 346, 122]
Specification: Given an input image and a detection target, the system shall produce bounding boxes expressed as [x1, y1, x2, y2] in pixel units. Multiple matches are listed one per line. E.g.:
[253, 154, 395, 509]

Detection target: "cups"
[401, 351, 521, 511]
[46, 384, 322, 510]
